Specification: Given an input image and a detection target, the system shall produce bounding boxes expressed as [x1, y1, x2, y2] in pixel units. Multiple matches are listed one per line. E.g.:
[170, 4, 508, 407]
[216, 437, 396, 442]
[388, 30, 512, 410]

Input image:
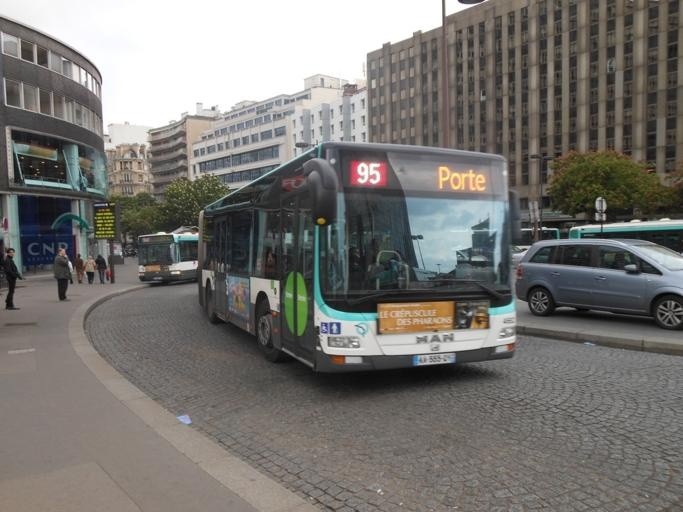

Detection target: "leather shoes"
[5, 300, 19, 310]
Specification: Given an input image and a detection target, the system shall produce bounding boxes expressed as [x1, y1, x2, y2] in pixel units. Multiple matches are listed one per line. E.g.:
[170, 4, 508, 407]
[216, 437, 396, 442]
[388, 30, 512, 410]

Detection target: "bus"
[195, 141, 516, 375]
[520, 227, 560, 240]
[570, 219, 682, 268]
[138, 226, 200, 283]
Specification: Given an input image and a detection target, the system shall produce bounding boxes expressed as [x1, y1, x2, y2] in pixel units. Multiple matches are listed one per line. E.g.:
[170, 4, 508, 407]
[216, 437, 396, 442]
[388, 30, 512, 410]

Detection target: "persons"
[63, 255, 74, 284]
[54, 248, 71, 301]
[3, 247, 22, 311]
[83, 255, 96, 284]
[74, 253, 84, 283]
[94, 253, 107, 284]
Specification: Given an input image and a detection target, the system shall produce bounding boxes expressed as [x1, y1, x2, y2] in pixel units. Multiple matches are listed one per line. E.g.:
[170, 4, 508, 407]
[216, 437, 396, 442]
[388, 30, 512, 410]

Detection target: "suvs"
[516, 238, 683, 331]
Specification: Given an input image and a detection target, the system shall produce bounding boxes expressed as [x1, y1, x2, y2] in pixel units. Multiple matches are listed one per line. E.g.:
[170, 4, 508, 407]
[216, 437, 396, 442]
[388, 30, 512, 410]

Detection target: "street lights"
[295, 142, 320, 149]
[531, 154, 555, 241]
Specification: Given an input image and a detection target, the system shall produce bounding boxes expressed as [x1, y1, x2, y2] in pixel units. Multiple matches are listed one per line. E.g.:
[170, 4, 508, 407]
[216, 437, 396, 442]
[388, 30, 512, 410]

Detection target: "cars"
[109, 252, 124, 264]
[124, 244, 138, 256]
[512, 245, 552, 275]
